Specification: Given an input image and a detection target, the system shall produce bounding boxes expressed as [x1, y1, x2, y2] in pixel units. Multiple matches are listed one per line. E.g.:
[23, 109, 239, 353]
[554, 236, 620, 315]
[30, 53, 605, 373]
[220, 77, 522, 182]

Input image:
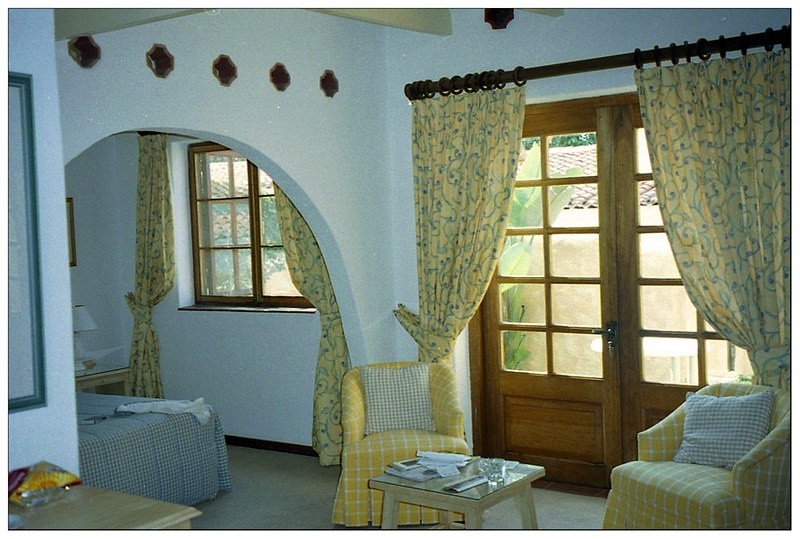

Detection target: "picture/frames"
[65, 197, 76, 266]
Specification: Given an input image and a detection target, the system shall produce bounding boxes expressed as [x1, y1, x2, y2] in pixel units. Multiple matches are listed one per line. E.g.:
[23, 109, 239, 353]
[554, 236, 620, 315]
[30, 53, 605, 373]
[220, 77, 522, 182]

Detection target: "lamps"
[72, 302, 98, 376]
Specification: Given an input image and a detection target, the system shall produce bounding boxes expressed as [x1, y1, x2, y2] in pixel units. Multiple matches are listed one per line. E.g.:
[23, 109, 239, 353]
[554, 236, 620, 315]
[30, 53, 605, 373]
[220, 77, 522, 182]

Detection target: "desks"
[8, 470, 204, 531]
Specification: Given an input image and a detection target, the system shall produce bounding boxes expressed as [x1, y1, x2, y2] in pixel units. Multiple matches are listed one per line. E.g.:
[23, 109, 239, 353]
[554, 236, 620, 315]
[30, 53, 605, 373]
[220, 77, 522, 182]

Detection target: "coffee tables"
[368, 451, 547, 530]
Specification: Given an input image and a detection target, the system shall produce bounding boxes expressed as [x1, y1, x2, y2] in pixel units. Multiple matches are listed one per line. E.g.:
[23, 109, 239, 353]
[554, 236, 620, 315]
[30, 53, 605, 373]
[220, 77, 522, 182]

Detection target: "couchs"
[595, 381, 791, 530]
[331, 361, 471, 528]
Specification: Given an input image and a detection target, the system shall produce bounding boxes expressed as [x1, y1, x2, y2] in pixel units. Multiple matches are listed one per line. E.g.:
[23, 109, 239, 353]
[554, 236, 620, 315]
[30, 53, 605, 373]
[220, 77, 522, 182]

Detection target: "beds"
[76, 390, 234, 508]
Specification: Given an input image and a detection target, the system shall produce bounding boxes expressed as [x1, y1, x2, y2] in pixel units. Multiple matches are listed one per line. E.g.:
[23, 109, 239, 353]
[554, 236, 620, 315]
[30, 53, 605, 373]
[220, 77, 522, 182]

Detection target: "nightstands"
[75, 365, 135, 396]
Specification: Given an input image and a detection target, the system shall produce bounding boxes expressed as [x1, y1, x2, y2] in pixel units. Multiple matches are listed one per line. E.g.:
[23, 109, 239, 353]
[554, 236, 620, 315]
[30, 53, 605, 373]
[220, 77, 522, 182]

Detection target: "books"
[384, 451, 481, 483]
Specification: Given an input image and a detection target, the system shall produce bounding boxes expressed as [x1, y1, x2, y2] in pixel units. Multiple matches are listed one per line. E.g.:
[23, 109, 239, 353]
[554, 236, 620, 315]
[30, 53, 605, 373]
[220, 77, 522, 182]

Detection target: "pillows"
[362, 363, 438, 436]
[671, 388, 778, 468]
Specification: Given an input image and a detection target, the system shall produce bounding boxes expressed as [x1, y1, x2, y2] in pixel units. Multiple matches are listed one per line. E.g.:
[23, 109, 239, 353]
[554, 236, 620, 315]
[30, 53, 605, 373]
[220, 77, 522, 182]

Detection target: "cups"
[487, 460, 506, 485]
